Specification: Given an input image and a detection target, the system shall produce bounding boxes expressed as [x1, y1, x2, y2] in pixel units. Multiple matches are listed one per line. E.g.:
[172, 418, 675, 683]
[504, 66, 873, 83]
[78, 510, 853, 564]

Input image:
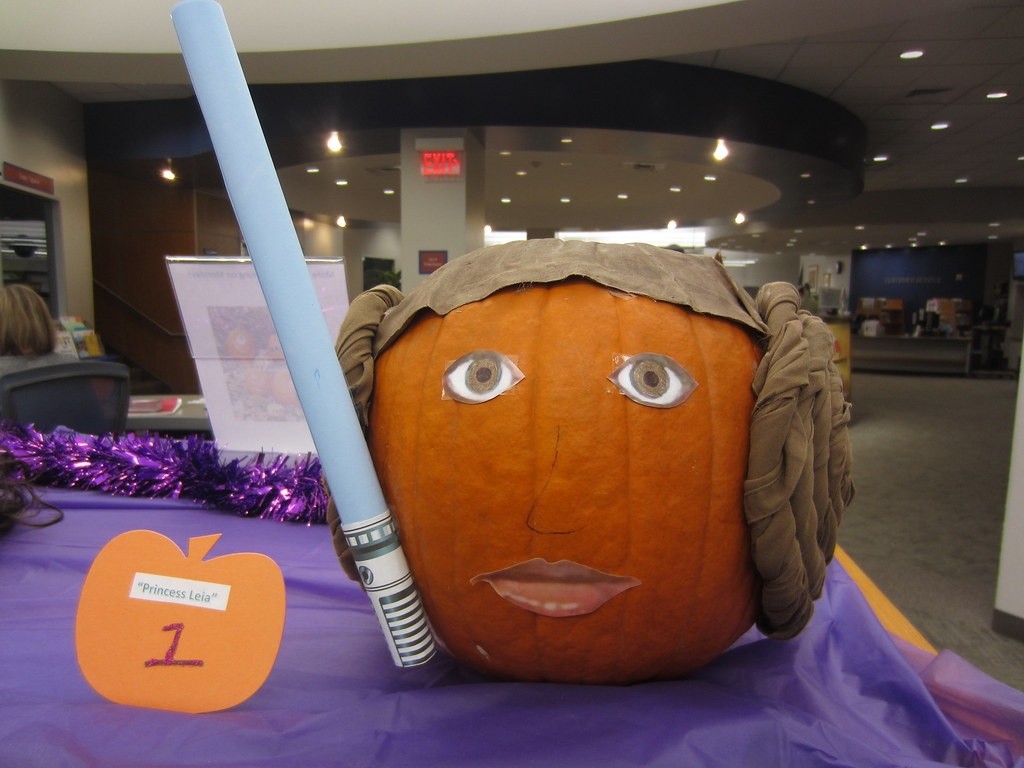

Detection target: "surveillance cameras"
[8, 245, 38, 259]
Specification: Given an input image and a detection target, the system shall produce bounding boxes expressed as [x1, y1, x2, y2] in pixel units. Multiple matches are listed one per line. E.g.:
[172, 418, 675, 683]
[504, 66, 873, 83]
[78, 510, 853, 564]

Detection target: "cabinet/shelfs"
[883, 298, 904, 335]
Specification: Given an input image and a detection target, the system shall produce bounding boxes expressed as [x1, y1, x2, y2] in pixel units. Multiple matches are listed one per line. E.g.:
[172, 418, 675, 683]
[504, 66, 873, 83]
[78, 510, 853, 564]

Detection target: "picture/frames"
[808, 264, 818, 293]
[823, 273, 830, 288]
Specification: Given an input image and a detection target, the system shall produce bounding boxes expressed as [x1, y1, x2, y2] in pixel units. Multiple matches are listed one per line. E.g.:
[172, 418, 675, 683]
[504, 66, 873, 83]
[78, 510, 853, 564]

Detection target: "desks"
[125, 393, 210, 430]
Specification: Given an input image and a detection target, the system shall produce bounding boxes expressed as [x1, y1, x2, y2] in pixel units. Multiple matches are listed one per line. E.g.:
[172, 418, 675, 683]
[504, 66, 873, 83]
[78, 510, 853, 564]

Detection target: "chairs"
[0, 363, 131, 435]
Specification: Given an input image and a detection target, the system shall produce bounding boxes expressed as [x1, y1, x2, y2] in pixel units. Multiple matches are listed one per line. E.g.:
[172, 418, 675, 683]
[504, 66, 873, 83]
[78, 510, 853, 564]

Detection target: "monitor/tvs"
[1012, 250, 1024, 281]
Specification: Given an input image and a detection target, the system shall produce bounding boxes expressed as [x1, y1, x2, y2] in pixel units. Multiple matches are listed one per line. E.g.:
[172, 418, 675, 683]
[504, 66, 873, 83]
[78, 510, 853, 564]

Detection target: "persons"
[1, 283, 80, 377]
